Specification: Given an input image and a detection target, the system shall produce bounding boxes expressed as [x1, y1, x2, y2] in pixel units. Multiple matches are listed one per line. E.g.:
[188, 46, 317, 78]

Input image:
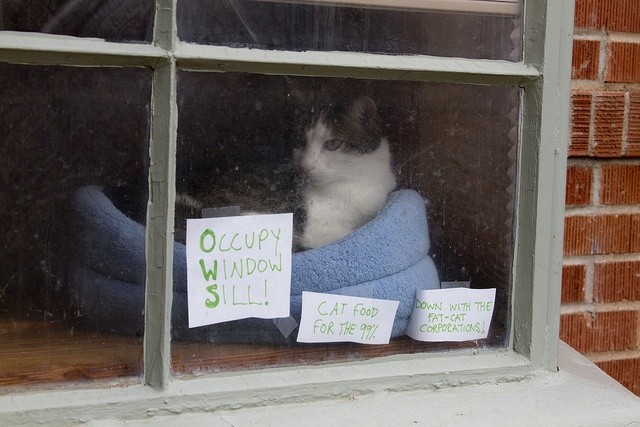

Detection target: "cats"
[102, 80, 397, 254]
